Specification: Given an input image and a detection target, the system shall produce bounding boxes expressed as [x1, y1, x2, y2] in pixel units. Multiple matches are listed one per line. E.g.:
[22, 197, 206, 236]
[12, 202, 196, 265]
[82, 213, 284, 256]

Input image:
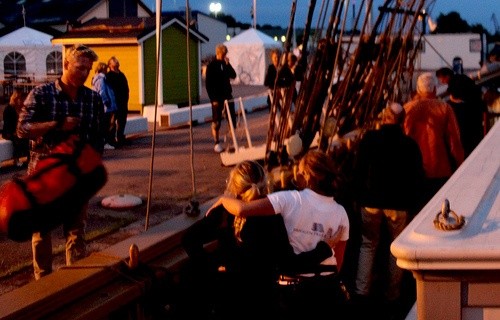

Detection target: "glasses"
[73, 46, 88, 55]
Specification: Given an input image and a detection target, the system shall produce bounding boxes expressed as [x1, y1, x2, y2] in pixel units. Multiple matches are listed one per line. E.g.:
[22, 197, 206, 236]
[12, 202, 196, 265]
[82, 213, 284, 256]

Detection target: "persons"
[2, 90, 31, 171]
[206, 150, 350, 320]
[342, 102, 428, 306]
[266, 39, 344, 129]
[104, 58, 132, 148]
[16, 45, 105, 280]
[478, 42, 500, 135]
[402, 73, 464, 191]
[181, 160, 345, 320]
[436, 68, 484, 160]
[206, 46, 237, 152]
[91, 62, 116, 149]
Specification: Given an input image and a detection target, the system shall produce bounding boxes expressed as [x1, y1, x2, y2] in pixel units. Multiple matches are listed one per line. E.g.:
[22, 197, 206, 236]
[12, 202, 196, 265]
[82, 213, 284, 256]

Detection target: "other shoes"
[104, 143, 116, 150]
[214, 143, 224, 153]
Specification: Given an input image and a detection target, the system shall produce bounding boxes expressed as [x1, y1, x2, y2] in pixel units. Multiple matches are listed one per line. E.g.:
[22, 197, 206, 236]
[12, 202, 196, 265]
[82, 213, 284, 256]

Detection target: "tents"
[222, 29, 286, 86]
[0, 27, 63, 82]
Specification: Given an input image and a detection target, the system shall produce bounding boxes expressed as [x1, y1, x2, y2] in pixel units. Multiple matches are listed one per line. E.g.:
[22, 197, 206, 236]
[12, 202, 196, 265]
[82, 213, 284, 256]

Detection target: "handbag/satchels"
[0, 132, 108, 242]
